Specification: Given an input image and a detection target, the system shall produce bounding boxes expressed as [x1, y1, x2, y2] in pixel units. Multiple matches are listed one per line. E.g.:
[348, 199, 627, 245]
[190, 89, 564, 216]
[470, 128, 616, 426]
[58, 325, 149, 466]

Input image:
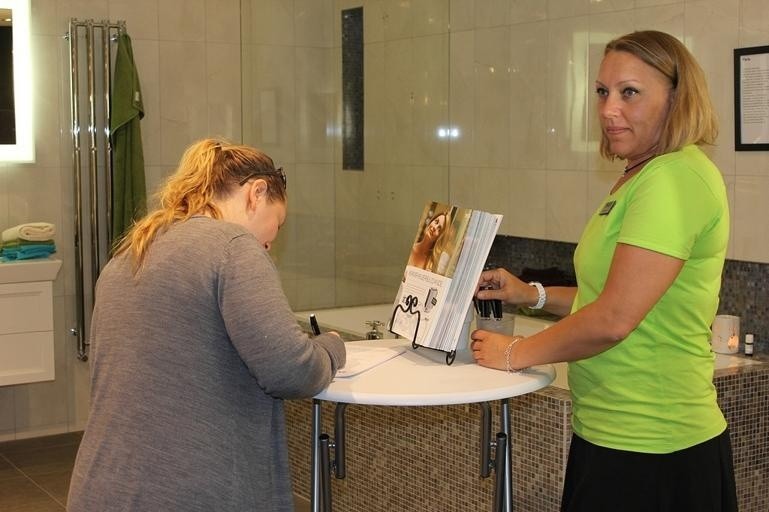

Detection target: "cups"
[475, 313, 515, 340]
[710, 314, 741, 354]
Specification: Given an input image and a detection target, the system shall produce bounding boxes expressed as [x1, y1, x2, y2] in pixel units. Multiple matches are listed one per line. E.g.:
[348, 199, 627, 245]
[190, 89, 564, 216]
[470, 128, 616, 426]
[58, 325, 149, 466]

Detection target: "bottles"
[744, 335, 754, 355]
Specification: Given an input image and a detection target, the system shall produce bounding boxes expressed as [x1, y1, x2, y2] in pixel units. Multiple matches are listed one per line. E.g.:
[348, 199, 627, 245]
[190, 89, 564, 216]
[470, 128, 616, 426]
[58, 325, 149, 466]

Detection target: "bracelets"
[504, 336, 523, 372]
[527, 282, 546, 310]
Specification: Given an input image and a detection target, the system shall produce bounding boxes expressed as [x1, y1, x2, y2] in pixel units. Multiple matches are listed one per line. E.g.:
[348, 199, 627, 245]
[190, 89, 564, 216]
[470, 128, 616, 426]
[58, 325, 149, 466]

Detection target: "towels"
[0, 222, 57, 262]
[110, 35, 147, 255]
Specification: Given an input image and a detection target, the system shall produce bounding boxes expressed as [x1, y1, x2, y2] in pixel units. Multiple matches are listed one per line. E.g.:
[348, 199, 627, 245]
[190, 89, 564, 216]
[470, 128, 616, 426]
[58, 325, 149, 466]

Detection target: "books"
[386, 200, 504, 355]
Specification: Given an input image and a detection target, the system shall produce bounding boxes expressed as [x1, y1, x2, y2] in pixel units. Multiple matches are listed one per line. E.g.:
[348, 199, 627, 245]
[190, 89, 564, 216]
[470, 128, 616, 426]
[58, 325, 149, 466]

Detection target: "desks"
[310, 338, 556, 511]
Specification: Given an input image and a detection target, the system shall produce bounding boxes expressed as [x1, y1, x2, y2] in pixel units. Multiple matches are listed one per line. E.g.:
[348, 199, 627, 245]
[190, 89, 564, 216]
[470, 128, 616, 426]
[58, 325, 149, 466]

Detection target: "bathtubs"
[282, 302, 769, 512]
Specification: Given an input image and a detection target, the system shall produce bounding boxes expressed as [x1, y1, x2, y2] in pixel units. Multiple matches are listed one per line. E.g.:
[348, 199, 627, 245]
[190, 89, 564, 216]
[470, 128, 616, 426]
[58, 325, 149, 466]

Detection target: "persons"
[65, 135, 347, 512]
[467, 29, 738, 512]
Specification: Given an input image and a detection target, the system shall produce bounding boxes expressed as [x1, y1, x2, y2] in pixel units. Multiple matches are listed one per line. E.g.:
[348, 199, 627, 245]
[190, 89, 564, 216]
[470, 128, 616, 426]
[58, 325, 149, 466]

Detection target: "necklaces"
[620, 153, 657, 178]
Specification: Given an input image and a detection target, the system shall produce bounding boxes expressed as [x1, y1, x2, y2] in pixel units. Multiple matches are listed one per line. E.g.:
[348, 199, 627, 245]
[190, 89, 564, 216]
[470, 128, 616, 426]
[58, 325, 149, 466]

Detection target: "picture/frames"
[734, 46, 768, 151]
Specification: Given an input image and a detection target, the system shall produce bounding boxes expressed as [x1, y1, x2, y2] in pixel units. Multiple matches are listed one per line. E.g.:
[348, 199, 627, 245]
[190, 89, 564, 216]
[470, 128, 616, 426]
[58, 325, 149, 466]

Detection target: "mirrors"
[1, 8, 17, 144]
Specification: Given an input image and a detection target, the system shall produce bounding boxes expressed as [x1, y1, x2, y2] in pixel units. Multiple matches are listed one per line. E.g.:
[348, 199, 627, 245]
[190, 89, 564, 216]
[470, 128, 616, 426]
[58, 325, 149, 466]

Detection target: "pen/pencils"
[310, 314, 321, 336]
[473, 285, 502, 318]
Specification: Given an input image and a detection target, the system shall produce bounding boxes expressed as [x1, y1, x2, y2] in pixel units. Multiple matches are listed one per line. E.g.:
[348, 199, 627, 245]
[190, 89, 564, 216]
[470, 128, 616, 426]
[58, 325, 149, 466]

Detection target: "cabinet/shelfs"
[0, 265, 66, 386]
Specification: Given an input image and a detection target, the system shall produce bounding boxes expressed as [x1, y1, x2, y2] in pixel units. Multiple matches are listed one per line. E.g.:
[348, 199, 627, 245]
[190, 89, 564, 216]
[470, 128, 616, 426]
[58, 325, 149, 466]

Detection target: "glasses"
[240, 167, 286, 189]
